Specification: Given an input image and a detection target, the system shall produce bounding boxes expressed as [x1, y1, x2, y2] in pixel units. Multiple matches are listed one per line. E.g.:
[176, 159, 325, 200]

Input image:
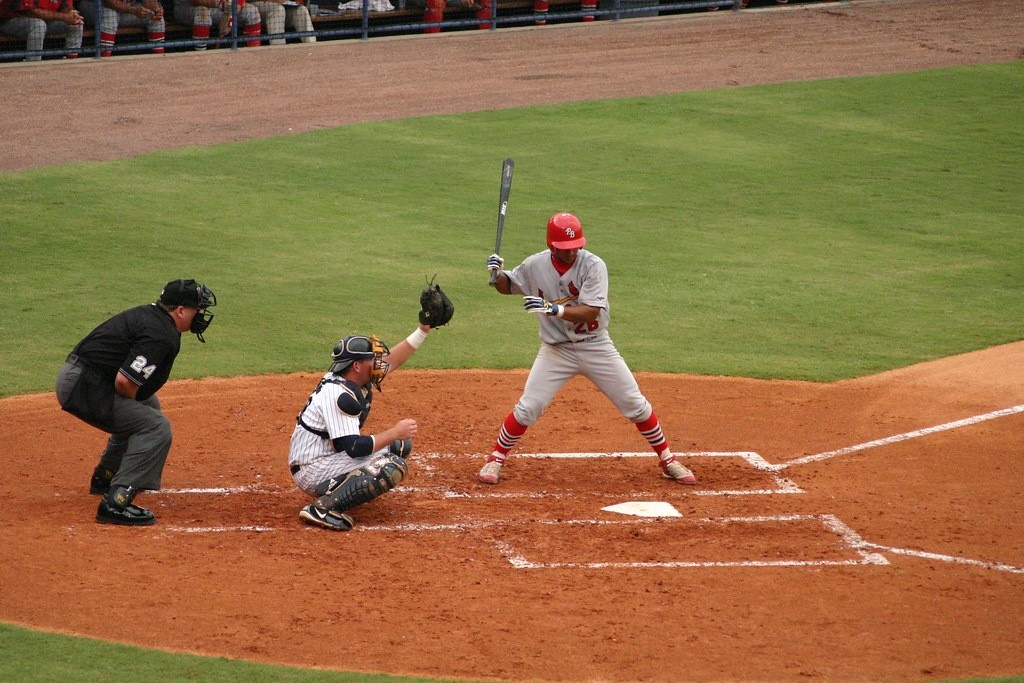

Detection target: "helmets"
[547, 212, 586, 254]
[331, 335, 374, 374]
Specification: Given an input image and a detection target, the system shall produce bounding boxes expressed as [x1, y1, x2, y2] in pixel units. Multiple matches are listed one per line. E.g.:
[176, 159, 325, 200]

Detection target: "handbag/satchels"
[62, 361, 116, 434]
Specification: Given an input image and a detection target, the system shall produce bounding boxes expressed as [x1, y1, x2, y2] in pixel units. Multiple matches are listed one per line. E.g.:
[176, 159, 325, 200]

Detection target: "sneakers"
[480, 454, 505, 484]
[299, 505, 353, 532]
[94, 494, 154, 526]
[658, 454, 697, 484]
[89, 467, 115, 494]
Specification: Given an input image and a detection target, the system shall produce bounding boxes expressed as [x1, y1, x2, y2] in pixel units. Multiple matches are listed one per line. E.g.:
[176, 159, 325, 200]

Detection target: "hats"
[161, 279, 212, 308]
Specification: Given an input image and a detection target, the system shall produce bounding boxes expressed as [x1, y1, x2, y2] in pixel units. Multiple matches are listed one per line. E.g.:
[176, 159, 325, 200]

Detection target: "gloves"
[523, 295, 558, 316]
[487, 254, 504, 279]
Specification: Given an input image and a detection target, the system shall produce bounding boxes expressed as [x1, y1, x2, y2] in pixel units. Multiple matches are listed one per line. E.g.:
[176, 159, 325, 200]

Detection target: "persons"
[478, 213, 697, 485]
[287, 272, 456, 531]
[0, 0, 661, 64]
[56, 278, 217, 526]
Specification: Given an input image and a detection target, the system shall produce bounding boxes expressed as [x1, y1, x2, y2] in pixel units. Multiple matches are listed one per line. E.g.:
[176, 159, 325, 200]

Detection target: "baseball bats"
[488, 158, 515, 287]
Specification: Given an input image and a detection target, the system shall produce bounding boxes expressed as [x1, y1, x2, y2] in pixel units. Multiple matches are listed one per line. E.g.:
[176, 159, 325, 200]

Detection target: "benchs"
[0, 0, 535, 50]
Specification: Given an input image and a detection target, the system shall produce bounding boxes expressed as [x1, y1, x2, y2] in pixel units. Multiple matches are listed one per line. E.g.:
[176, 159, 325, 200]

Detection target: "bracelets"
[405, 326, 428, 351]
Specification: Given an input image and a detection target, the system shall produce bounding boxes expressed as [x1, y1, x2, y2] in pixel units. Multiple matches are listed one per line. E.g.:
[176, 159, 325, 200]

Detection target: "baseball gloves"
[417, 272, 455, 331]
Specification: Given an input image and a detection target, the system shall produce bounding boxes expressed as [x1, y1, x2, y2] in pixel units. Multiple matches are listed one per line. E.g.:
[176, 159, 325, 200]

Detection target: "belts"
[564, 336, 597, 343]
[290, 465, 300, 475]
[65, 353, 86, 369]
